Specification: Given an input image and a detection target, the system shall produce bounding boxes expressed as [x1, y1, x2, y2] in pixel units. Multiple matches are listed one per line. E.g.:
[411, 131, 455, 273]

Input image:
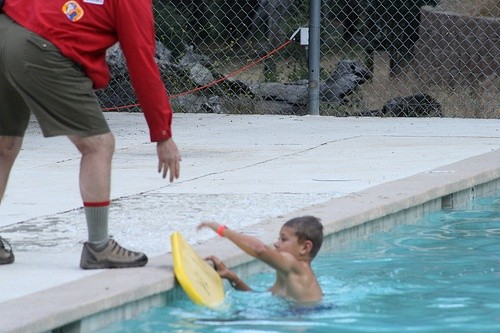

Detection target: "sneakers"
[0, 236, 14, 265]
[80, 238, 148, 269]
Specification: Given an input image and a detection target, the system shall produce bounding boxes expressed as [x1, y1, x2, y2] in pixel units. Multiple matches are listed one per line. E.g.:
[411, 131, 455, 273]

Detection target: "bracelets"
[218, 225, 228, 237]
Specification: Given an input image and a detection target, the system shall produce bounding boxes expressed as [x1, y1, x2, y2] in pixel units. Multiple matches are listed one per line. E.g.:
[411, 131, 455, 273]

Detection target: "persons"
[0, 0, 184, 269]
[197, 215, 326, 301]
